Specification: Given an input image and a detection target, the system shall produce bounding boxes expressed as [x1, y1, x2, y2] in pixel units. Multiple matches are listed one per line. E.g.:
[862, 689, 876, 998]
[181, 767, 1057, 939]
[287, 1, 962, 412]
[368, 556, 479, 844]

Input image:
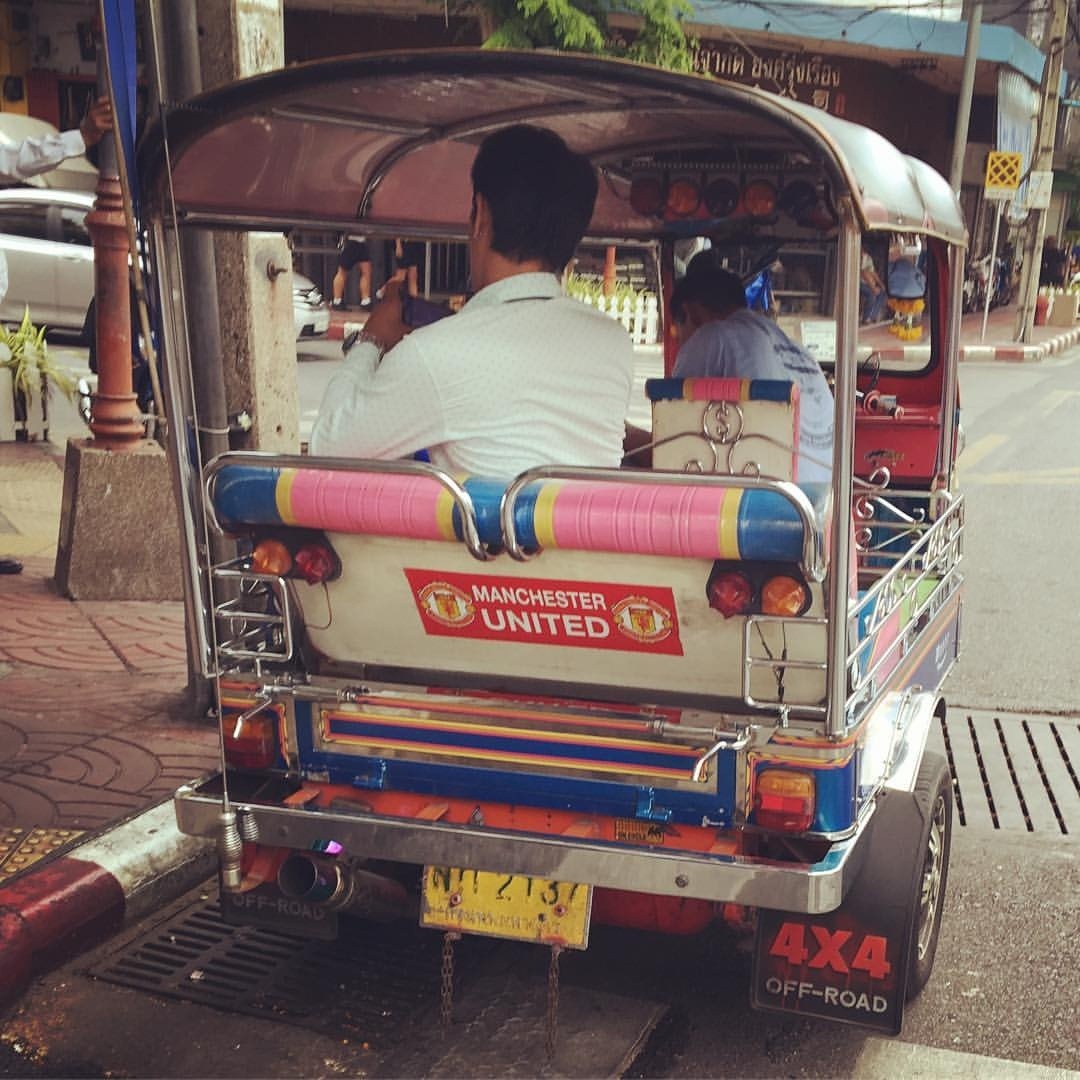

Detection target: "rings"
[107, 104, 111, 112]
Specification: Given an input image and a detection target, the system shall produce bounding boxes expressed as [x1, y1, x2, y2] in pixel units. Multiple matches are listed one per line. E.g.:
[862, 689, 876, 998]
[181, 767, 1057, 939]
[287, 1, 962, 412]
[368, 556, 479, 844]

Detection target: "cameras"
[400, 295, 453, 326]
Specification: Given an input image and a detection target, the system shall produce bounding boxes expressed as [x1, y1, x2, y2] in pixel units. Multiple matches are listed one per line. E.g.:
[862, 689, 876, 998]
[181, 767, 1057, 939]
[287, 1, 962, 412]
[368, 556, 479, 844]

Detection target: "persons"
[620, 249, 835, 483]
[333, 236, 371, 310]
[859, 246, 887, 320]
[376, 240, 420, 302]
[0, 95, 115, 575]
[309, 124, 633, 485]
[1044, 235, 1062, 273]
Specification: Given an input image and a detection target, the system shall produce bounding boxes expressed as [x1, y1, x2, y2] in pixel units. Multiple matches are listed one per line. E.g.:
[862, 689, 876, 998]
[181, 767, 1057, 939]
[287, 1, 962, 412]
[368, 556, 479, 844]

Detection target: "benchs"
[202, 449, 828, 706]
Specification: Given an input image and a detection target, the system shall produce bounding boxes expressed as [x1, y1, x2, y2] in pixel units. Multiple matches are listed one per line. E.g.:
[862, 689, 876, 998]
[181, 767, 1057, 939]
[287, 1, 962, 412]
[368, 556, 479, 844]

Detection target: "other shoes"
[331, 300, 353, 311]
[359, 302, 376, 311]
